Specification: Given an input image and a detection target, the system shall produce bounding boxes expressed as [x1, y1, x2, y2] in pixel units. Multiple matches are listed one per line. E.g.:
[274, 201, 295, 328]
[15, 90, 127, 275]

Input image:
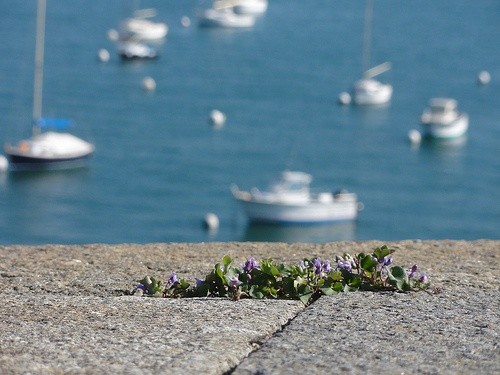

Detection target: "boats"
[354, 79, 392, 104]
[115, 17, 168, 59]
[5, 132, 96, 161]
[420, 98, 468, 139]
[237, 170, 358, 224]
[203, 2, 254, 27]
